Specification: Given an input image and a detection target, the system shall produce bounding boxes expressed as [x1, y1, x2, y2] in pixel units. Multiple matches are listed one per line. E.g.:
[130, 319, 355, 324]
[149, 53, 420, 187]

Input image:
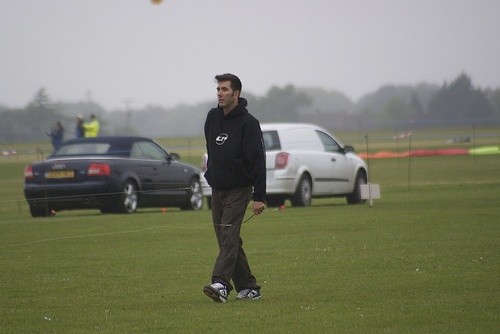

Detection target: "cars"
[23, 136, 204, 217]
[199, 122, 368, 210]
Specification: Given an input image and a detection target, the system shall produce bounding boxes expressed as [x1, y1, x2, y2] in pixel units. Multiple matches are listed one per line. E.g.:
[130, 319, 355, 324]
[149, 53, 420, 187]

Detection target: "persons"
[46, 121, 64, 149]
[203, 73, 267, 302]
[82, 114, 100, 138]
[75, 115, 85, 138]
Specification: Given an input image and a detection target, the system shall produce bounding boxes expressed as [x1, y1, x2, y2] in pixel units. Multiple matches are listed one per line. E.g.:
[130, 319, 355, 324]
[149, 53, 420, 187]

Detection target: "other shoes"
[203, 283, 228, 303]
[236, 288, 263, 301]
